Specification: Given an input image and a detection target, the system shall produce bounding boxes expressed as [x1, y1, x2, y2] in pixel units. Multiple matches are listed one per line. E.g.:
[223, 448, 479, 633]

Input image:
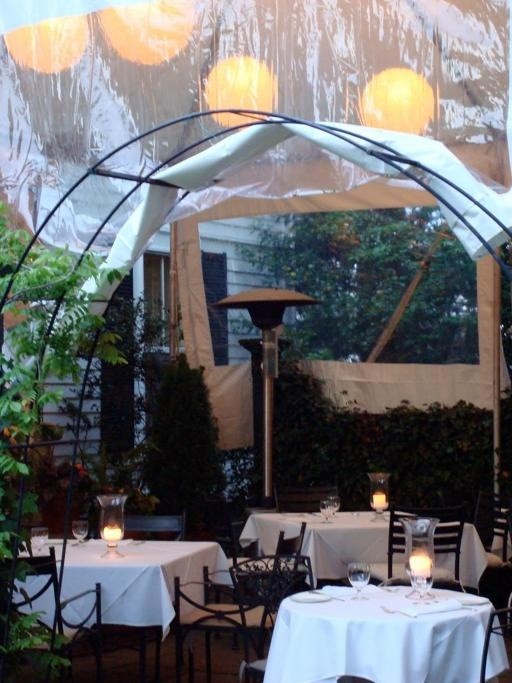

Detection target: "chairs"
[475, 497, 512, 587]
[477, 604, 512, 683]
[349, 506, 466, 592]
[225, 485, 281, 558]
[227, 554, 316, 683]
[173, 520, 307, 683]
[84, 503, 186, 683]
[0, 545, 104, 683]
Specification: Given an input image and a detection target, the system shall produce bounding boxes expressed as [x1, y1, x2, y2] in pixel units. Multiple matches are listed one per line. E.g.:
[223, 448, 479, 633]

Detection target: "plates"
[460, 596, 489, 606]
[291, 593, 331, 604]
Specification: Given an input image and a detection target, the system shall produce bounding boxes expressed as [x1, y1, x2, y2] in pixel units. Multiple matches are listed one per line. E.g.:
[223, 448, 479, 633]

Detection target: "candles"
[373, 494, 386, 505]
[409, 554, 433, 573]
[103, 527, 121, 538]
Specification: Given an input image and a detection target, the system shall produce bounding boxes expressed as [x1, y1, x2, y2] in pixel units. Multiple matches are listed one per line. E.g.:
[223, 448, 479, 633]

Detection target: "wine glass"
[319, 496, 341, 524]
[97, 493, 129, 560]
[72, 520, 89, 548]
[398, 517, 441, 605]
[348, 563, 370, 594]
[367, 472, 391, 523]
[32, 528, 49, 552]
